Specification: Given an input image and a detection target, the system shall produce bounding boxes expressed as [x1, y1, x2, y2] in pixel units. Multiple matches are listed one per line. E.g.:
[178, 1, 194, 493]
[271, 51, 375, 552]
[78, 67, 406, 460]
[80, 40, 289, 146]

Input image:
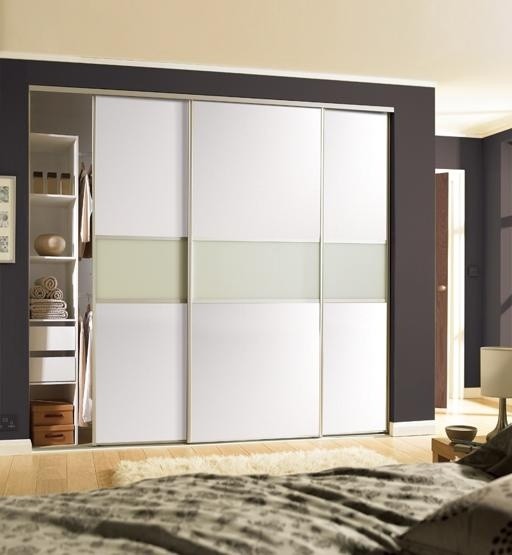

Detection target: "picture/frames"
[0, 175, 16, 264]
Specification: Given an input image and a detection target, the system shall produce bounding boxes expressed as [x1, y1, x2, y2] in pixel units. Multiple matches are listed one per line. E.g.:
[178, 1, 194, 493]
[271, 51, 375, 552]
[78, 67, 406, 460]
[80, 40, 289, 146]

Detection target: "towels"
[30, 277, 68, 319]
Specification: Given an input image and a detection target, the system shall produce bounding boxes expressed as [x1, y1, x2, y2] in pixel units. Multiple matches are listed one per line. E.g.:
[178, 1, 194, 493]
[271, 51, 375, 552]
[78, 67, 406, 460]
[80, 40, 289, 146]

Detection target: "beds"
[0, 461, 496, 555]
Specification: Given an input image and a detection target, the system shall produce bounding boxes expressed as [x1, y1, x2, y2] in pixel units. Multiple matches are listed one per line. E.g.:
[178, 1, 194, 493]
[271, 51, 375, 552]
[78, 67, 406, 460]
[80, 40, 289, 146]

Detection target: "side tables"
[432, 436, 490, 463]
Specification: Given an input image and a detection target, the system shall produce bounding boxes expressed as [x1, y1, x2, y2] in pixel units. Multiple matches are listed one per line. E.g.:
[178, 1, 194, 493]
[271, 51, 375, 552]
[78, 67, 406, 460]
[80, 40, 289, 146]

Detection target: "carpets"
[112, 446, 401, 488]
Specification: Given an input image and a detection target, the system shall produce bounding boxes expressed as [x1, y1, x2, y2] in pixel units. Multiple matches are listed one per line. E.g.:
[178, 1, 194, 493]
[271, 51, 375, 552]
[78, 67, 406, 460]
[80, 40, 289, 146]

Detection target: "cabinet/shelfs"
[28, 131, 82, 447]
[28, 86, 393, 449]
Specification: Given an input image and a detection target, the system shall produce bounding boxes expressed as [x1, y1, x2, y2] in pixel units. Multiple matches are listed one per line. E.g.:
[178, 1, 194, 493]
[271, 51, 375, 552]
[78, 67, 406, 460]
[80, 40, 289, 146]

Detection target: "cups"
[59, 173, 72, 195]
[32, 171, 44, 194]
[47, 172, 57, 194]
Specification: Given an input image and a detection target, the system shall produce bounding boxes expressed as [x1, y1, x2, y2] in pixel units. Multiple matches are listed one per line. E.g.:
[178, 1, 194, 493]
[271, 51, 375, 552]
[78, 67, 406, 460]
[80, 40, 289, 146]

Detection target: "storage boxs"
[34, 425, 73, 445]
[32, 398, 73, 426]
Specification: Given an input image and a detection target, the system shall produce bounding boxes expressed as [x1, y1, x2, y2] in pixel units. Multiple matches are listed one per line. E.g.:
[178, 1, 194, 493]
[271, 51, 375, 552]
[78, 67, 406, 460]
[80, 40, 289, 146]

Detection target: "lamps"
[480, 347, 512, 441]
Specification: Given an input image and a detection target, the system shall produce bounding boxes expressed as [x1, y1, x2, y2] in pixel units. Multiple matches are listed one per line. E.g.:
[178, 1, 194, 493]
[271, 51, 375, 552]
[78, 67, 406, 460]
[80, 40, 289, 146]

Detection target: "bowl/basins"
[445, 425, 478, 442]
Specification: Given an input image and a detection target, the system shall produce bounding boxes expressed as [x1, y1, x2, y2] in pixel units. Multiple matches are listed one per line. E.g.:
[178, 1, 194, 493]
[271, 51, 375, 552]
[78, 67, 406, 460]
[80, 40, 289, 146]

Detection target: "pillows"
[395, 473, 512, 555]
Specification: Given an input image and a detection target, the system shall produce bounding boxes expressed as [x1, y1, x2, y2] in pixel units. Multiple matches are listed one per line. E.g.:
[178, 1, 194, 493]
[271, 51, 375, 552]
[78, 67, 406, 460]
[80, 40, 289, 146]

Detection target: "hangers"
[81, 152, 86, 171]
